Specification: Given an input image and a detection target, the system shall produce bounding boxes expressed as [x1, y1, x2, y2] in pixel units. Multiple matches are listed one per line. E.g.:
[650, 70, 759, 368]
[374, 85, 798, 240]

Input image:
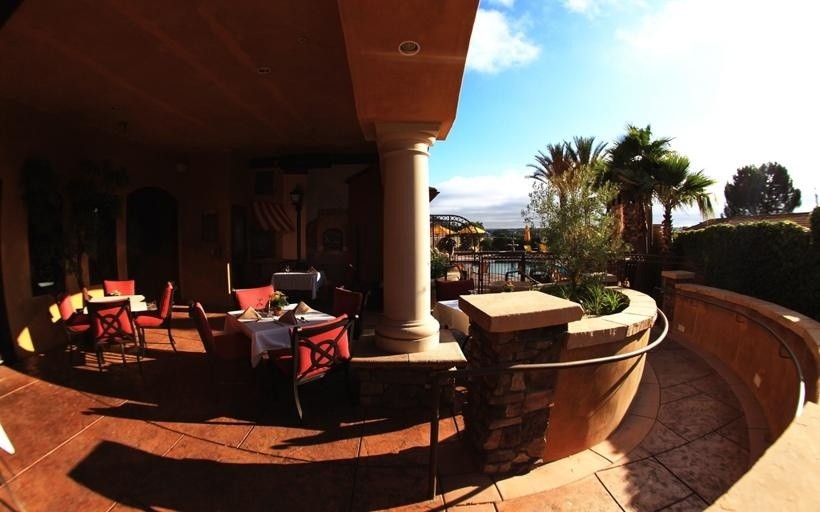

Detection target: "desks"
[437, 300, 472, 352]
[82, 294, 150, 346]
[224, 302, 337, 387]
[271, 269, 326, 310]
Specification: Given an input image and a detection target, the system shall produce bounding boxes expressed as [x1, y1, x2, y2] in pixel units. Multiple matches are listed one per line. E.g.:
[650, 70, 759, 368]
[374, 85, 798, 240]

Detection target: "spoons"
[301, 317, 310, 323]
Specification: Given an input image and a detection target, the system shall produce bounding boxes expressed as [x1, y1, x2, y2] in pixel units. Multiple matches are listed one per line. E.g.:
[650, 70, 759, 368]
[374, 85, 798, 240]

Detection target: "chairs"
[190, 300, 264, 405]
[104, 279, 136, 296]
[331, 287, 364, 357]
[435, 277, 475, 301]
[231, 284, 277, 309]
[134, 281, 179, 357]
[58, 289, 105, 363]
[83, 298, 144, 376]
[275, 313, 353, 426]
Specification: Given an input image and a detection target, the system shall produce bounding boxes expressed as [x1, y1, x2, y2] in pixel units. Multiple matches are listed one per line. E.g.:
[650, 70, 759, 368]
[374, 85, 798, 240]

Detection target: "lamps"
[289, 189, 305, 213]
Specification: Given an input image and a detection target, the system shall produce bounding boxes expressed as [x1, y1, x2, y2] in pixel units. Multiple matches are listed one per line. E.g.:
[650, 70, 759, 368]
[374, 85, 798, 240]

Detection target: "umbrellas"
[430, 222, 459, 248]
[459, 225, 486, 247]
[523, 225, 530, 243]
